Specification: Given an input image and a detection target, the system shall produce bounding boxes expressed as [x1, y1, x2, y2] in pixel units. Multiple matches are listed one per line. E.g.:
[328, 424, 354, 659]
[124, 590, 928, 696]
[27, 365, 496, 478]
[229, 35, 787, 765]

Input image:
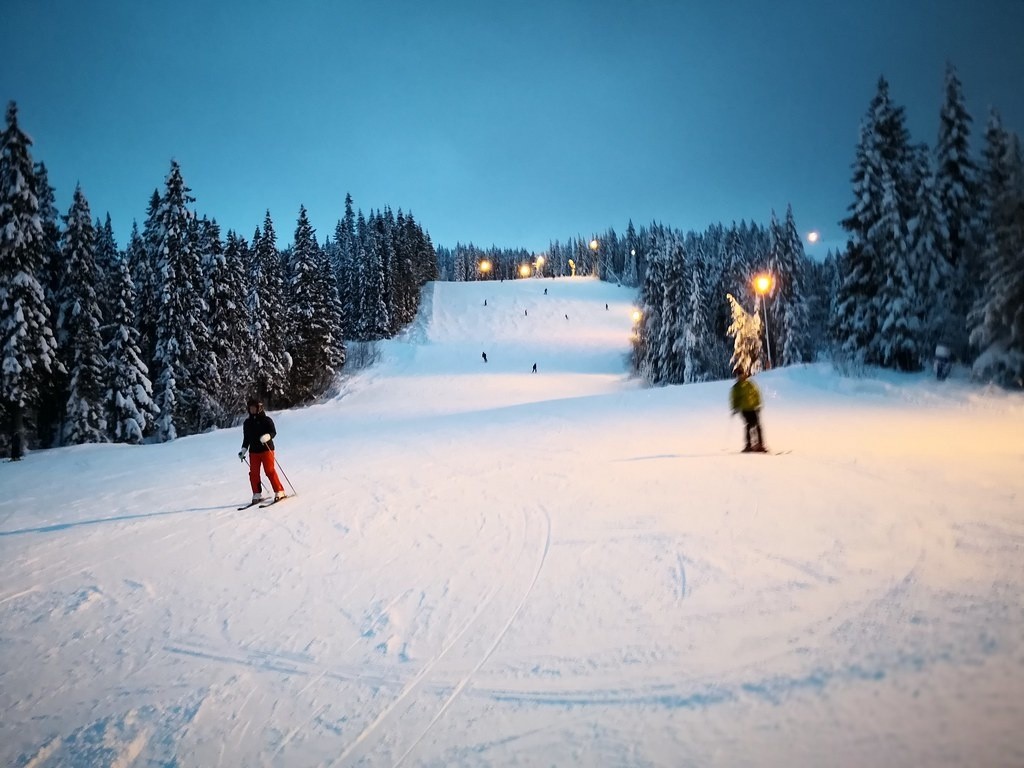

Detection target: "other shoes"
[252, 493, 264, 502]
[275, 490, 284, 501]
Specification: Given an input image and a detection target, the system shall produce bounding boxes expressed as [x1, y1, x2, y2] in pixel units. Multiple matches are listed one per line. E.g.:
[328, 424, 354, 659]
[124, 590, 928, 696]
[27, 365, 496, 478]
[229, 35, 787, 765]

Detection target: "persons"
[730, 367, 768, 453]
[501, 277, 503, 283]
[532, 363, 538, 373]
[238, 400, 285, 502]
[606, 304, 609, 311]
[524, 310, 528, 316]
[482, 352, 488, 363]
[544, 288, 547, 295]
[484, 299, 487, 305]
[564, 315, 568, 319]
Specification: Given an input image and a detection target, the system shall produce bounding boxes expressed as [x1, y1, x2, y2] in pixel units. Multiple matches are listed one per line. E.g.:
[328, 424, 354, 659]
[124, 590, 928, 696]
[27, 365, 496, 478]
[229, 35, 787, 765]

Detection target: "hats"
[247, 399, 259, 408]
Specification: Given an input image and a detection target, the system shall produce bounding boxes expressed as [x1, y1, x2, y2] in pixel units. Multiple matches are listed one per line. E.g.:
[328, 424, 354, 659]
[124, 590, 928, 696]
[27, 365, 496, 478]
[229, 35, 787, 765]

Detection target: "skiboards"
[237, 493, 295, 511]
[776, 450, 792, 455]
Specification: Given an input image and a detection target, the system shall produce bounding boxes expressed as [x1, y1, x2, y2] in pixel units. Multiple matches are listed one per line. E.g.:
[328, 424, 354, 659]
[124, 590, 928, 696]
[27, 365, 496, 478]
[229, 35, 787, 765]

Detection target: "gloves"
[239, 448, 248, 463]
[260, 434, 271, 444]
